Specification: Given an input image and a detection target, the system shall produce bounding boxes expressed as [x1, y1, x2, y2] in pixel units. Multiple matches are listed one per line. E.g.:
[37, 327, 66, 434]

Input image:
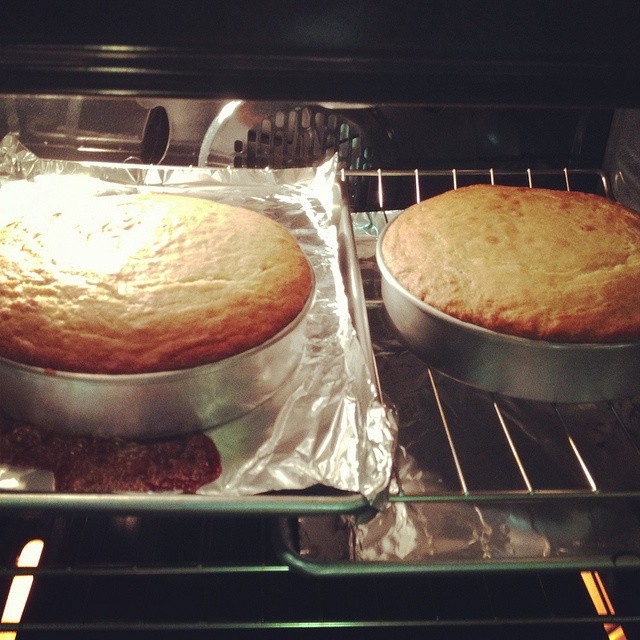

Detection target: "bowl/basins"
[1, 250, 318, 445]
[375, 209, 640, 404]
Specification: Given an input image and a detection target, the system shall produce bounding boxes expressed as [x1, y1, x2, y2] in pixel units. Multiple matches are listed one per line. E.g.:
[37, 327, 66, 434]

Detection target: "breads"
[380, 184, 639, 344]
[0, 192, 314, 374]
